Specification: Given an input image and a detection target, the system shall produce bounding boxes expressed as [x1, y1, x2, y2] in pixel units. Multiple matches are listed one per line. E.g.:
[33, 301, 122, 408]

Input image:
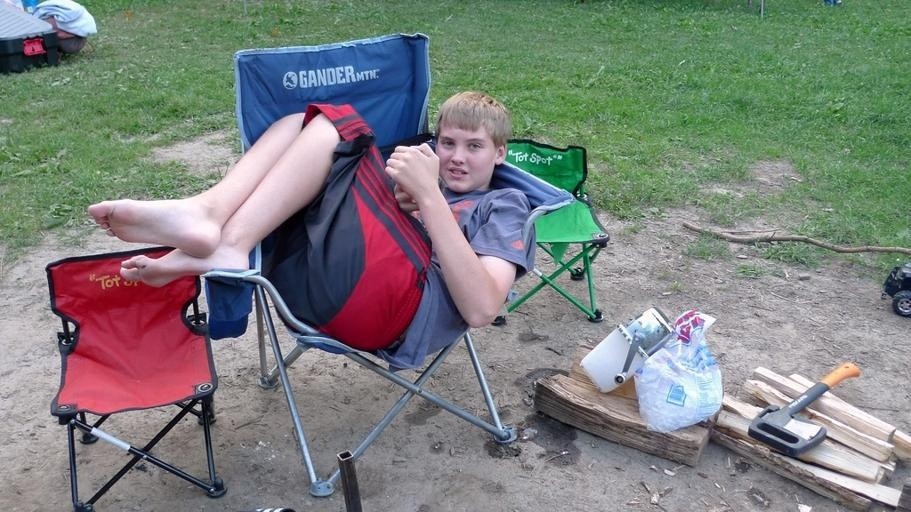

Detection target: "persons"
[87, 92, 536, 369]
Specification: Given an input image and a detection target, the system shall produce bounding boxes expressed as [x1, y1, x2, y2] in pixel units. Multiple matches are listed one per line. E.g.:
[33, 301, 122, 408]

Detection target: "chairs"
[495, 138, 608, 324]
[200, 33, 573, 496]
[46, 245, 226, 511]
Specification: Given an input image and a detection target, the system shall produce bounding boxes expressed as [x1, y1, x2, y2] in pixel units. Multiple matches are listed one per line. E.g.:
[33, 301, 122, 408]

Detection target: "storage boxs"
[0, 32, 59, 72]
